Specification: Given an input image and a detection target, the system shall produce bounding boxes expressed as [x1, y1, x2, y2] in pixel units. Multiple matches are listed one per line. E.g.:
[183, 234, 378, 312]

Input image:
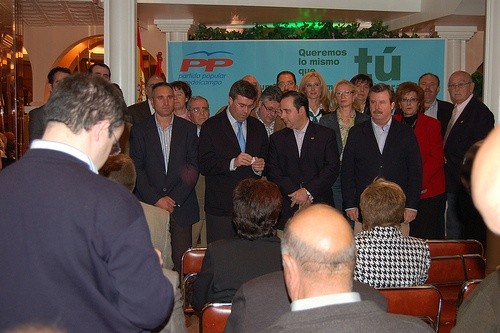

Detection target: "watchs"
[304, 187, 310, 196]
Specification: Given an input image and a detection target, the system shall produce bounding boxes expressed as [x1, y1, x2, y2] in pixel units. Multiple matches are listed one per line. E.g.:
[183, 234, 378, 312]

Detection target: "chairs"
[181, 240, 486, 333]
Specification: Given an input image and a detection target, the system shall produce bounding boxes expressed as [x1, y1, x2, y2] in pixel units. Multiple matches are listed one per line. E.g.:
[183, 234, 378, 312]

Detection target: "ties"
[236, 121, 246, 154]
[444, 106, 458, 143]
[309, 109, 321, 123]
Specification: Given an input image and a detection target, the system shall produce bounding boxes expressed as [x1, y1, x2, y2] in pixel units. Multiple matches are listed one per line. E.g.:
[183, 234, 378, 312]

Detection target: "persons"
[197, 71, 341, 243]
[320, 72, 446, 239]
[256, 204, 436, 333]
[352, 177, 431, 289]
[190, 179, 283, 312]
[443, 71, 495, 258]
[98, 155, 175, 270]
[451, 126, 500, 333]
[0, 72, 175, 333]
[123, 75, 210, 288]
[28, 64, 126, 148]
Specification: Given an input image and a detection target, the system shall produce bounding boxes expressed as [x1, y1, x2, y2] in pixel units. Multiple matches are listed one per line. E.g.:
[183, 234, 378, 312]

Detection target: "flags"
[137, 22, 144, 83]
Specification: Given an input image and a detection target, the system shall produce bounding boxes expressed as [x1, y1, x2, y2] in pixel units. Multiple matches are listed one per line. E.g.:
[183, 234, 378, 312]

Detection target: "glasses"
[190, 108, 207, 113]
[449, 82, 470, 89]
[401, 98, 417, 103]
[335, 90, 354, 96]
[109, 130, 121, 157]
[262, 103, 279, 115]
